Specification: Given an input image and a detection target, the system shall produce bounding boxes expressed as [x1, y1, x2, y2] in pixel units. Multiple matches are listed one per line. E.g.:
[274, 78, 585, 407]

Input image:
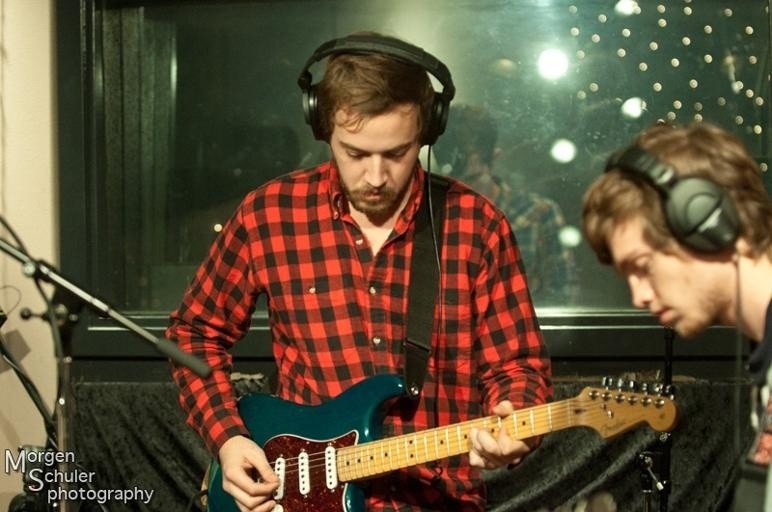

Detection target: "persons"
[581, 121, 772, 512]
[162, 30, 553, 512]
[433, 101, 580, 307]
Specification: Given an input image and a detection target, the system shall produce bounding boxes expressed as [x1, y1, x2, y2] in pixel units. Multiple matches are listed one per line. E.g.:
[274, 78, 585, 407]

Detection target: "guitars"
[207, 372, 676, 512]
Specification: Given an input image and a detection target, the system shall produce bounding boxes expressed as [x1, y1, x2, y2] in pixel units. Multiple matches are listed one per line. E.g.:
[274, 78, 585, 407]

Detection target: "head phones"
[604, 144, 742, 255]
[297, 36, 455, 145]
